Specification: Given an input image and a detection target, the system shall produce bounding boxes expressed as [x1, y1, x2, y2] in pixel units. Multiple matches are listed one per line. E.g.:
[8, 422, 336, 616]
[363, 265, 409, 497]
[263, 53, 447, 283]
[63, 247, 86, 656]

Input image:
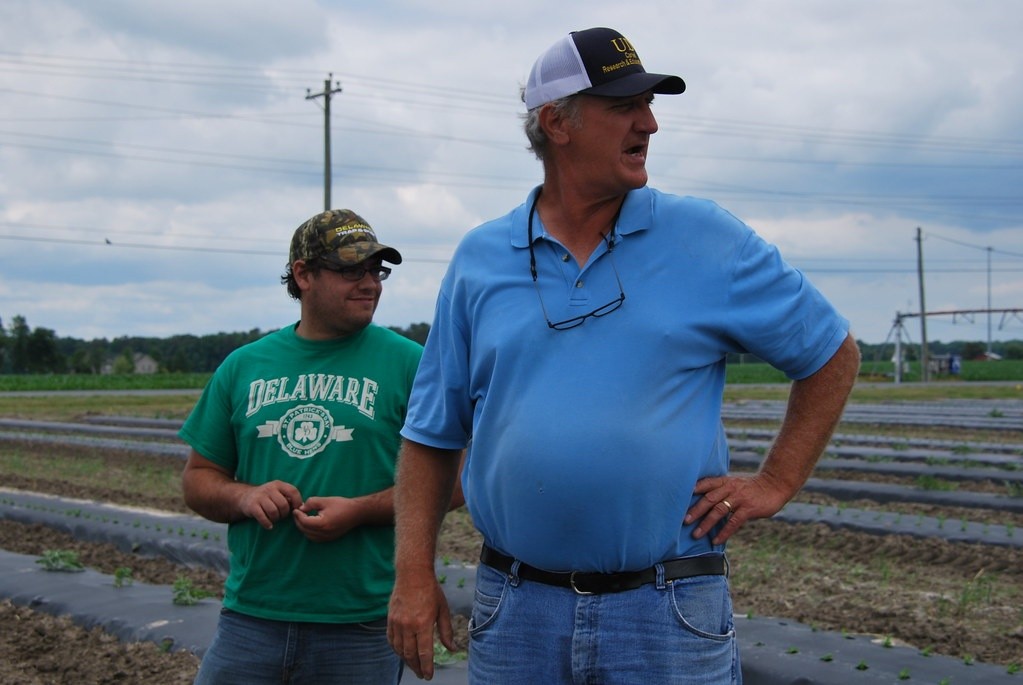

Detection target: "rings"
[722, 500, 733, 512]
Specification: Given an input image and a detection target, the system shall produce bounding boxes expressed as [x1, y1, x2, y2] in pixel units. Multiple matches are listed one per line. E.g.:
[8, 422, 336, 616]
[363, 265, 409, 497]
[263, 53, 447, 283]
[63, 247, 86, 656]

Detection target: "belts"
[477, 542, 728, 596]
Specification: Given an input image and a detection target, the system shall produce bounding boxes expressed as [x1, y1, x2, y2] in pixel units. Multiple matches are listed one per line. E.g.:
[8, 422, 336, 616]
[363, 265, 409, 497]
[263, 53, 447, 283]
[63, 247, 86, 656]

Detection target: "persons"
[176, 209, 466, 685]
[386, 27, 861, 685]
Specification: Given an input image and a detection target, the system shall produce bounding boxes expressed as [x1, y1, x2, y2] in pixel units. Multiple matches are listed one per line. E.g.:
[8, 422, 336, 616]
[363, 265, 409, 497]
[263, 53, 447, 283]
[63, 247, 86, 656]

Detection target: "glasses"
[315, 264, 392, 282]
[527, 230, 627, 332]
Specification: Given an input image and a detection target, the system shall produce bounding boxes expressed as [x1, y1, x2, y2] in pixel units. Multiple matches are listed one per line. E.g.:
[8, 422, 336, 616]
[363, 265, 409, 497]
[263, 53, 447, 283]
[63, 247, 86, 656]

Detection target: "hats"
[288, 208, 402, 267]
[524, 26, 686, 112]
